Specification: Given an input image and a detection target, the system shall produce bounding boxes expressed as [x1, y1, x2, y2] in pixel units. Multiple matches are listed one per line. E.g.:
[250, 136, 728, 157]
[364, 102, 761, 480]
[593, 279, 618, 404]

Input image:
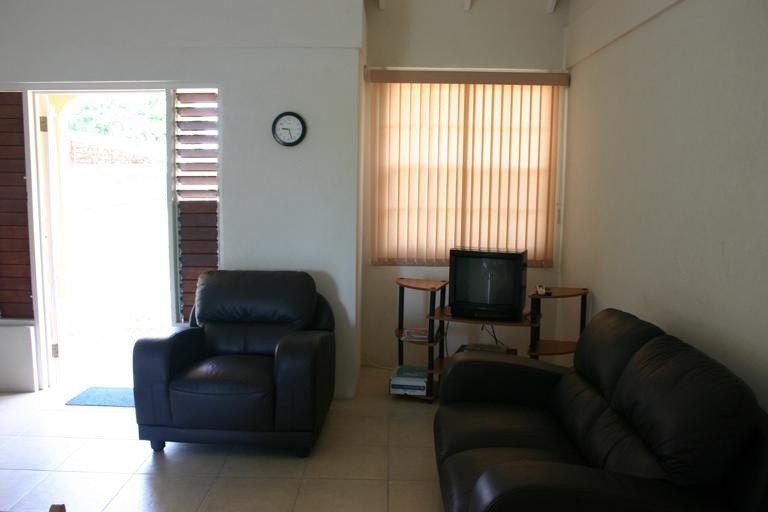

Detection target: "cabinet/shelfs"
[395, 277, 591, 403]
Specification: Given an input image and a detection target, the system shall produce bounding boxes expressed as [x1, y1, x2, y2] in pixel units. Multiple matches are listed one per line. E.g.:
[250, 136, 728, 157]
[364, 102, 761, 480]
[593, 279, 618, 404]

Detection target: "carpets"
[64, 385, 136, 407]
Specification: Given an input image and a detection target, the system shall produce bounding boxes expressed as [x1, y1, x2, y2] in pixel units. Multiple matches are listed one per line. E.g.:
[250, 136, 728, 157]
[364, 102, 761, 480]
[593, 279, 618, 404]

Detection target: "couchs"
[433, 307, 768, 512]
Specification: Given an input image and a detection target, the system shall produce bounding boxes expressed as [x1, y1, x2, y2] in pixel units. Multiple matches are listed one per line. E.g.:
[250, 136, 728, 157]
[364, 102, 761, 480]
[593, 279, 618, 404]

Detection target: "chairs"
[132, 269, 337, 457]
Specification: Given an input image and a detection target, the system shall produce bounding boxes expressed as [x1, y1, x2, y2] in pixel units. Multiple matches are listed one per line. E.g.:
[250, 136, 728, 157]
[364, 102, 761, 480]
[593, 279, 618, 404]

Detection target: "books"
[404, 328, 428, 342]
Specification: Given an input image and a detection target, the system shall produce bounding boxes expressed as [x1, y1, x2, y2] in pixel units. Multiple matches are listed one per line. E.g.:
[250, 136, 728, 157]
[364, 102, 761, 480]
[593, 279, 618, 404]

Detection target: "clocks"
[271, 112, 308, 147]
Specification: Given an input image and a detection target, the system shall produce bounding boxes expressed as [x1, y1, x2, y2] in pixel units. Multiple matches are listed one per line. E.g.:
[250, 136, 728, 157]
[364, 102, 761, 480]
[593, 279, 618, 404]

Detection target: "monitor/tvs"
[449, 246, 528, 323]
